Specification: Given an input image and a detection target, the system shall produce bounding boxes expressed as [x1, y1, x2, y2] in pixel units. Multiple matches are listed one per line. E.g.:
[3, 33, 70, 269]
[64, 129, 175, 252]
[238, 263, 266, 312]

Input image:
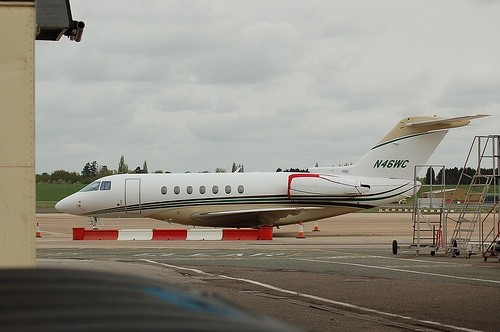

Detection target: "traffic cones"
[311, 218, 319, 230]
[294, 220, 306, 240]
[35, 222, 41, 237]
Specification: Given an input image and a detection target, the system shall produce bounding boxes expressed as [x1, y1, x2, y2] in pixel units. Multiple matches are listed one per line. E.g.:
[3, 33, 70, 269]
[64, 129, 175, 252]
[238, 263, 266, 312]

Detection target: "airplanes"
[54, 113, 490, 231]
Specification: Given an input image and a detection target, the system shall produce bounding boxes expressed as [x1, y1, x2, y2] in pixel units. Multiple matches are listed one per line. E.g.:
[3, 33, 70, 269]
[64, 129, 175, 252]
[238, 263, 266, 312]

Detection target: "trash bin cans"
[415, 191, 445, 209]
